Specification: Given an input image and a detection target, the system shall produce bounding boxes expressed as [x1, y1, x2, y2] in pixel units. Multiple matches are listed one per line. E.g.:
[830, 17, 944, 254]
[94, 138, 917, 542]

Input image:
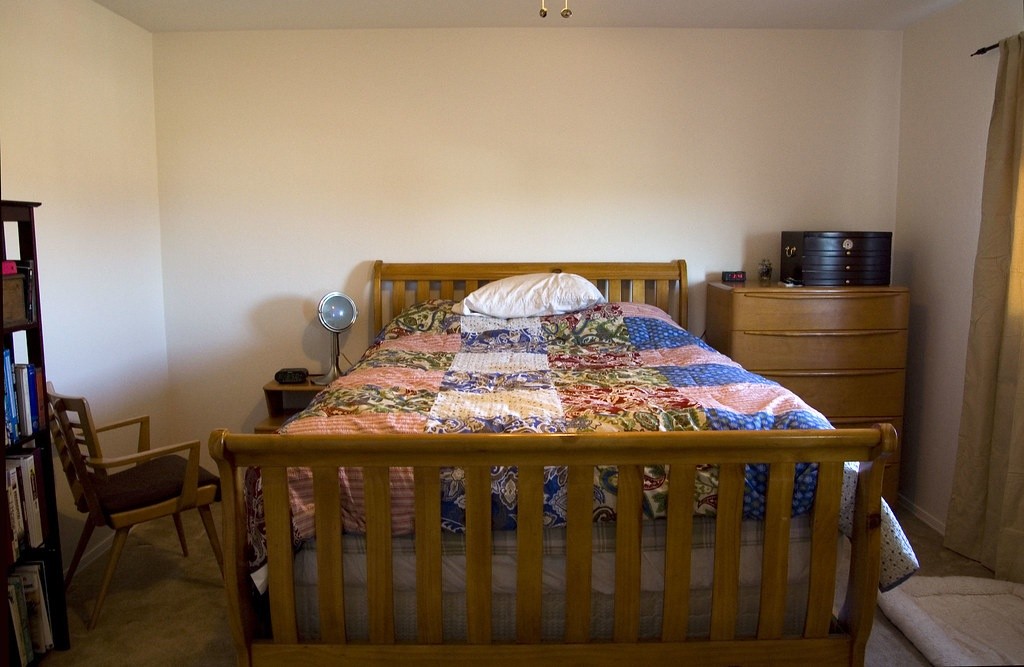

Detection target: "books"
[3, 350, 38, 445]
[19, 258, 36, 324]
[6, 455, 46, 561]
[7, 566, 55, 664]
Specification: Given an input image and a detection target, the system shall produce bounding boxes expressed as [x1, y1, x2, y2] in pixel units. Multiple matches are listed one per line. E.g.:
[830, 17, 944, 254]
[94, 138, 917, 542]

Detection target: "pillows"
[452, 273, 608, 319]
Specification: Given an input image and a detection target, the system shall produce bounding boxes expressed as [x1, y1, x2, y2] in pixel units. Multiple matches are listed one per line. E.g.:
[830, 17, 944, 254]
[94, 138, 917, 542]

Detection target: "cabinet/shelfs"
[0, 200, 71, 667]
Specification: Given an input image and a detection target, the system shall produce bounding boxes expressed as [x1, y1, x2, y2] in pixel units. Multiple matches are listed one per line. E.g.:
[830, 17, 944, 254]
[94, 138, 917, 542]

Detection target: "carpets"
[876, 576, 1024, 667]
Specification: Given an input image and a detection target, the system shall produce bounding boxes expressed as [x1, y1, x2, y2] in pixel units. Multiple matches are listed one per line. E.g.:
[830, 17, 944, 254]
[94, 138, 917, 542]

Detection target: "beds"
[206, 259, 921, 667]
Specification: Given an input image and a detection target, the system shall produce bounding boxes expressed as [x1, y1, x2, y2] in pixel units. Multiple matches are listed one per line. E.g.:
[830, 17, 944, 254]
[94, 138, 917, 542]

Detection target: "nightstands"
[706, 279, 910, 506]
[253, 374, 327, 433]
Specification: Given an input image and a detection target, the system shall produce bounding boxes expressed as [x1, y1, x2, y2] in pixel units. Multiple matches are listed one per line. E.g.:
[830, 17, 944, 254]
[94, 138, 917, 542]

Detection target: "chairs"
[44, 381, 224, 631]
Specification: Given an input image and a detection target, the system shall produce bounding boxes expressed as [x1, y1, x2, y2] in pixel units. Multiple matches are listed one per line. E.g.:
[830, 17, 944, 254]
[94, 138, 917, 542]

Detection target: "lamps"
[313, 291, 358, 386]
